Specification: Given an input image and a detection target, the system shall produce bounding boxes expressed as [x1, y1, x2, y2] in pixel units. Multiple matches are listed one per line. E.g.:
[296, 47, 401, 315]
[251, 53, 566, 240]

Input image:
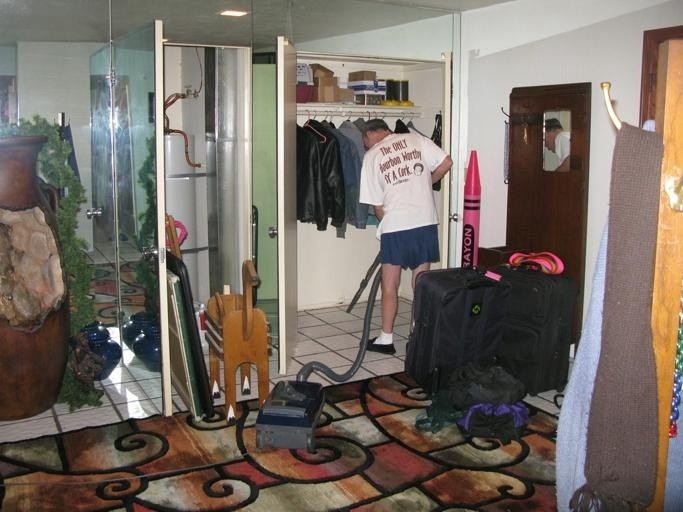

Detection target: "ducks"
[403, 263, 575, 403]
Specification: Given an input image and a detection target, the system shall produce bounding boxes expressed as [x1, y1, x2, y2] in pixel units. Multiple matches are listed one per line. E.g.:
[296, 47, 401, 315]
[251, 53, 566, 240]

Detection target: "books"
[311, 64, 385, 106]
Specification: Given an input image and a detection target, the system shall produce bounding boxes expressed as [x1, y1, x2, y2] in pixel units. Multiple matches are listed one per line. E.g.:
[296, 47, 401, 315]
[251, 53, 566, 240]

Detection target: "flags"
[0, 135, 70, 421]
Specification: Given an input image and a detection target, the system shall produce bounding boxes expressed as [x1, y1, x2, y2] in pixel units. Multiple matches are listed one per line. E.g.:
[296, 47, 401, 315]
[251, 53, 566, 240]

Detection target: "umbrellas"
[360, 336, 397, 355]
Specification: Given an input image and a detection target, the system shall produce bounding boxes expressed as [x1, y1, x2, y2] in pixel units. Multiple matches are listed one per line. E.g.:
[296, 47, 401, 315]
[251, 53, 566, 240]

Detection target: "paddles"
[0, 372, 559, 512]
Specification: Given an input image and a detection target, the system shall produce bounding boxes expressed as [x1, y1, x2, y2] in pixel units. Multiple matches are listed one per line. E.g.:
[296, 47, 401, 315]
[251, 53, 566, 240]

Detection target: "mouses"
[296, 109, 415, 144]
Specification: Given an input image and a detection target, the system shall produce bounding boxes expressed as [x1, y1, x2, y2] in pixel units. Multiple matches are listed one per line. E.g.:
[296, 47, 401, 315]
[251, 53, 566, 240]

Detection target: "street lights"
[413, 355, 532, 443]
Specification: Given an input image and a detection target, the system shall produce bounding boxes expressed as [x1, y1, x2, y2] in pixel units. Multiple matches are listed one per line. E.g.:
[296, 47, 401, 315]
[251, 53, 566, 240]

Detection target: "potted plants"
[165, 249, 215, 422]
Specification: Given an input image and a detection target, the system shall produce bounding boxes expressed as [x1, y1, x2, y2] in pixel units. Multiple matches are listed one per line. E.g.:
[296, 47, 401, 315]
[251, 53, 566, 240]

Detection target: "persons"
[543, 118, 570, 173]
[358, 117, 452, 353]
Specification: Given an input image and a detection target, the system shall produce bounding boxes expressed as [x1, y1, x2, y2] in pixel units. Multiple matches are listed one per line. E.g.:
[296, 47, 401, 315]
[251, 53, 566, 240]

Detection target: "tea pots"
[542, 109, 572, 173]
